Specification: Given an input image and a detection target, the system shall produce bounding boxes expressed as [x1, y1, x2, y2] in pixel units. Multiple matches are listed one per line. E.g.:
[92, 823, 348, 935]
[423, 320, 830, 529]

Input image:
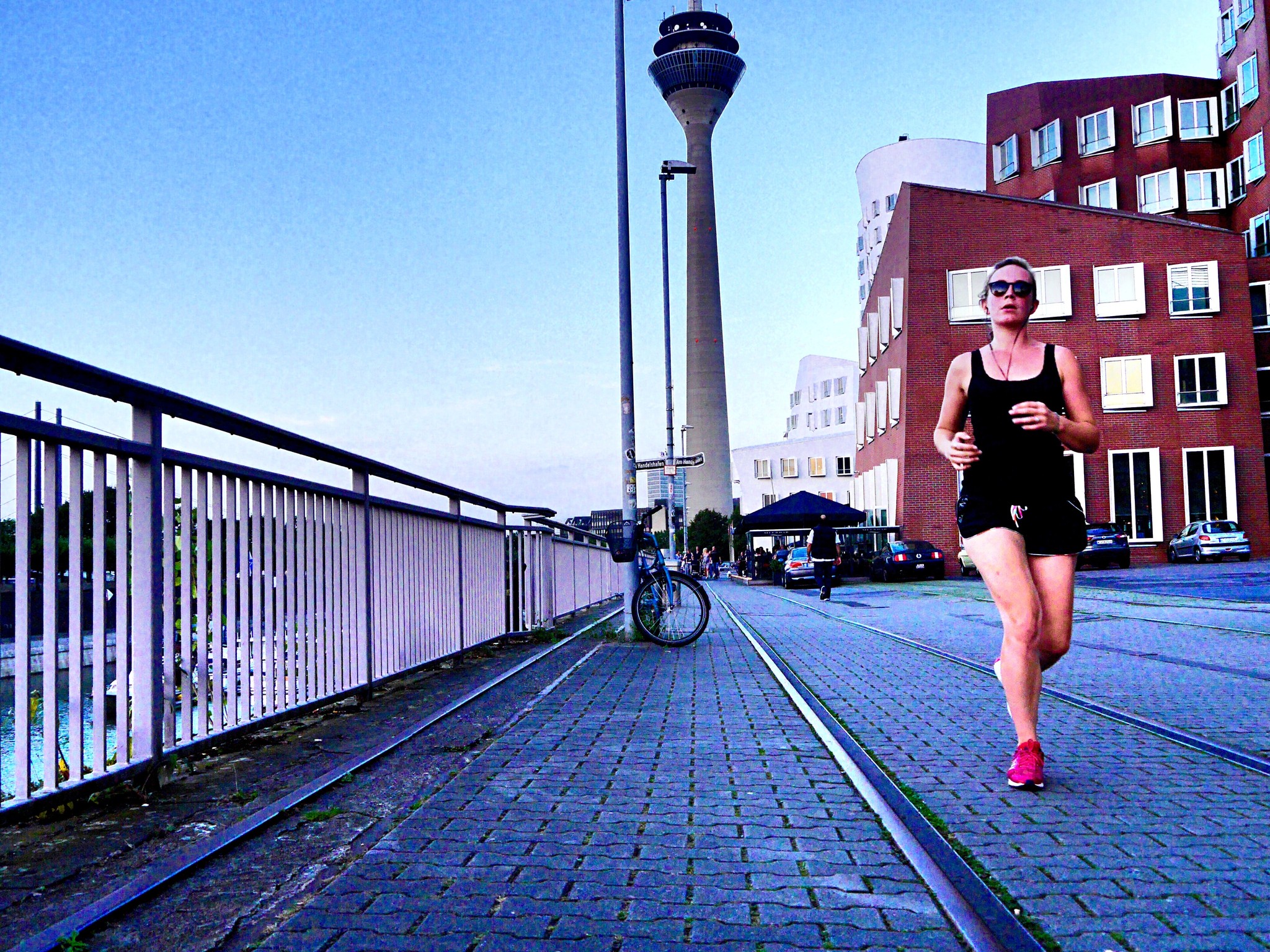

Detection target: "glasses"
[989, 281, 1032, 297]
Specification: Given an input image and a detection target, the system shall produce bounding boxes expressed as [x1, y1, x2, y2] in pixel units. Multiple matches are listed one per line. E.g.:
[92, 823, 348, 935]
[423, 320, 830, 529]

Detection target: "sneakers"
[821, 598, 830, 602]
[1008, 741, 1050, 790]
[819, 585, 825, 599]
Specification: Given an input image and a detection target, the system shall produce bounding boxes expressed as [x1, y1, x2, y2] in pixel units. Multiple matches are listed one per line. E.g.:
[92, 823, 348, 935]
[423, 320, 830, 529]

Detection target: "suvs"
[1075, 522, 1130, 569]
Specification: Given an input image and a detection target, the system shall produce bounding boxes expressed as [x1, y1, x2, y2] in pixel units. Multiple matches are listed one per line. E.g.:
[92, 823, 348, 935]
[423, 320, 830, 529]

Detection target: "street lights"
[682, 425, 694, 575]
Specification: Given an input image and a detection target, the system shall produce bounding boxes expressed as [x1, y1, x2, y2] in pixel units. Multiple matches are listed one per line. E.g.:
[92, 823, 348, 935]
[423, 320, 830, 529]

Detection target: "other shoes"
[702, 574, 720, 579]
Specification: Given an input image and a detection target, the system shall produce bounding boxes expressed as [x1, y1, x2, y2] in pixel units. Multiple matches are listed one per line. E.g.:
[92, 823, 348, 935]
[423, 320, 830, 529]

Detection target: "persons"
[931, 256, 1101, 791]
[807, 514, 841, 602]
[676, 546, 721, 578]
[738, 537, 788, 578]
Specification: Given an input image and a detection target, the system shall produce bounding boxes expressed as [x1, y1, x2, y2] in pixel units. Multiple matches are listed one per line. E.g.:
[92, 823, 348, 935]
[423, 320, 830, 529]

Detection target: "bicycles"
[605, 504, 711, 647]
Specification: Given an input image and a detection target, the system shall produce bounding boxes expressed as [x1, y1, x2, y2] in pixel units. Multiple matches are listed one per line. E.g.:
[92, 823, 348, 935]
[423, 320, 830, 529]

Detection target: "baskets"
[605, 518, 642, 563]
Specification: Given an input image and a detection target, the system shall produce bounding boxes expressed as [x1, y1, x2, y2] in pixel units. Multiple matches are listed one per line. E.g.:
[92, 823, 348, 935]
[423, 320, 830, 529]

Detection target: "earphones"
[985, 306, 989, 314]
[1030, 304, 1034, 312]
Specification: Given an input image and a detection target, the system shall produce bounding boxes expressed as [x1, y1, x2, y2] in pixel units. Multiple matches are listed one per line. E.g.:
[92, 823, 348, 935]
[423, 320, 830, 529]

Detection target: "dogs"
[727, 571, 732, 579]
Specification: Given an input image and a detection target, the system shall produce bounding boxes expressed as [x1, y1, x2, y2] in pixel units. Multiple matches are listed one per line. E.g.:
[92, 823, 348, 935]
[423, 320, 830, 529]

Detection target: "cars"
[1168, 520, 1250, 562]
[958, 545, 982, 577]
[782, 547, 838, 590]
[867, 540, 946, 581]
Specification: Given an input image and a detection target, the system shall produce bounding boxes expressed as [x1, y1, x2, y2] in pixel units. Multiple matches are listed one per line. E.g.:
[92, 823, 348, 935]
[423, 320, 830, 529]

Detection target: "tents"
[732, 491, 867, 549]
